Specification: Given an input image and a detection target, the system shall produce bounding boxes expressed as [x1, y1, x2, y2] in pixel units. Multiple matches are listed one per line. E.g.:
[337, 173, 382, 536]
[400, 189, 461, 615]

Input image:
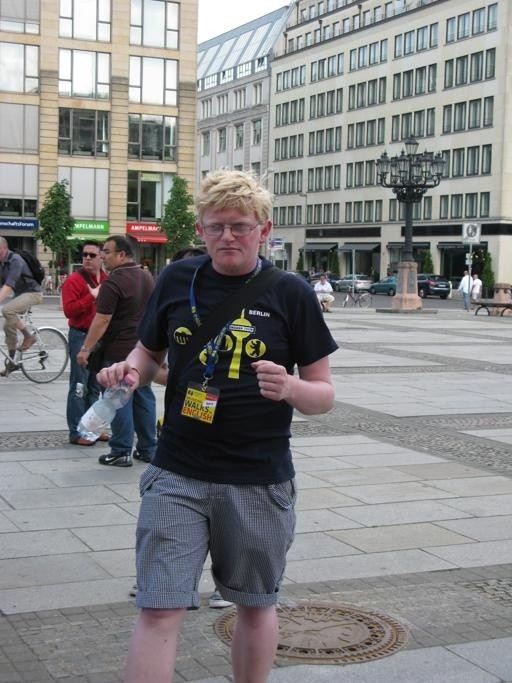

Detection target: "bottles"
[75, 371, 139, 444]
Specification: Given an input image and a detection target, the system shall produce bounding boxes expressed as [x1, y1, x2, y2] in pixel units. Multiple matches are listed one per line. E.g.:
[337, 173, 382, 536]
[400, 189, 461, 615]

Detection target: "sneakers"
[99, 453, 132, 467]
[209, 590, 234, 608]
[17, 336, 37, 350]
[0, 362, 20, 376]
[98, 433, 111, 441]
[133, 450, 150, 463]
[71, 436, 95, 445]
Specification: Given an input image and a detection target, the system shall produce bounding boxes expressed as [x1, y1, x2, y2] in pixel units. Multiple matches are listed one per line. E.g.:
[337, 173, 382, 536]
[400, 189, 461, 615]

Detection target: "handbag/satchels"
[86, 347, 103, 372]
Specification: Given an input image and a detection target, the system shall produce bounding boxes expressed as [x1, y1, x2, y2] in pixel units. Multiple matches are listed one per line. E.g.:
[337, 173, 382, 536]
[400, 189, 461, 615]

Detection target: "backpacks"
[7, 249, 44, 286]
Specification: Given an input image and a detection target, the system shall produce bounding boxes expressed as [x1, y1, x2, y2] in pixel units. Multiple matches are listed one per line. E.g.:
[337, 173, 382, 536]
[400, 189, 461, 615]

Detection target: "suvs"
[416, 272, 450, 298]
[335, 273, 374, 293]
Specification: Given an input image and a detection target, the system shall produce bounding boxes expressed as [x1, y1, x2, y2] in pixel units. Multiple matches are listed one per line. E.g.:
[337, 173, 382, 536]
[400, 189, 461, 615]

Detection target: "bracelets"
[81, 345, 91, 352]
[131, 366, 141, 388]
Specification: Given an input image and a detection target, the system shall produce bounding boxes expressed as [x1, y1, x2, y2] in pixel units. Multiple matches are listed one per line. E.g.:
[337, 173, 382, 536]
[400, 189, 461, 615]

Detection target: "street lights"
[374, 132, 446, 312]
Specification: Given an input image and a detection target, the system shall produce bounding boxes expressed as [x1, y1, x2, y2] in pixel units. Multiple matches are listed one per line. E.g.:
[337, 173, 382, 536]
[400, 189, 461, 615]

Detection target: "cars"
[370, 275, 398, 296]
[287, 270, 310, 284]
[308, 271, 339, 289]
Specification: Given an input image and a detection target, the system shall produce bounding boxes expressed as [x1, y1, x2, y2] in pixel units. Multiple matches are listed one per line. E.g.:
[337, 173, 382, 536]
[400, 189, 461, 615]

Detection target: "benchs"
[470, 299, 512, 318]
[320, 299, 328, 312]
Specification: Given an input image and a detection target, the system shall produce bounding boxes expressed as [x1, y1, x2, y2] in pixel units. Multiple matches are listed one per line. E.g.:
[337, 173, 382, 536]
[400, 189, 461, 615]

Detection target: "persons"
[313, 275, 335, 312]
[471, 274, 482, 309]
[128, 249, 234, 608]
[0, 237, 43, 376]
[78, 236, 158, 467]
[457, 271, 473, 310]
[62, 240, 111, 446]
[96, 169, 338, 683]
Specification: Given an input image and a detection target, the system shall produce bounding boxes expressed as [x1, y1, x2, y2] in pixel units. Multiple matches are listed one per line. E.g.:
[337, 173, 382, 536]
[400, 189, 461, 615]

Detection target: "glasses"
[82, 252, 99, 258]
[202, 222, 259, 237]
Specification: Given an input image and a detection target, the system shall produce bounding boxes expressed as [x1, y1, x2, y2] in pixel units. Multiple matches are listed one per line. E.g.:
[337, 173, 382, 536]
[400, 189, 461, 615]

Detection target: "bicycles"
[340, 284, 374, 311]
[1, 304, 72, 384]
